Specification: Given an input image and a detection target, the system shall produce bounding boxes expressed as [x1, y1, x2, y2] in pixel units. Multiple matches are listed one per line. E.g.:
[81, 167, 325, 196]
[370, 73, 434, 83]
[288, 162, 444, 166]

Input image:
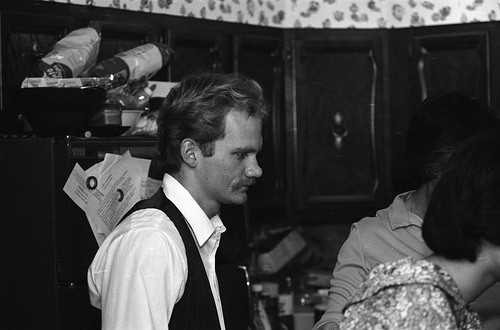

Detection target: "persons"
[339, 129, 500, 330]
[87, 65, 271, 330]
[312, 91, 500, 330]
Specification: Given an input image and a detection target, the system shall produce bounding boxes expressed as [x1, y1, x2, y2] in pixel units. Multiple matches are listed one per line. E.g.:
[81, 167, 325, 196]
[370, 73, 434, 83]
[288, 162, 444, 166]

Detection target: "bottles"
[252, 284, 264, 316]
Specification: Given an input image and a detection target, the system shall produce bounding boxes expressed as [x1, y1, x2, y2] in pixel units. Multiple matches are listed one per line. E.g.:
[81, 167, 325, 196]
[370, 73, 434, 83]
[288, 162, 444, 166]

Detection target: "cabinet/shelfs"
[0, 0, 500, 226]
